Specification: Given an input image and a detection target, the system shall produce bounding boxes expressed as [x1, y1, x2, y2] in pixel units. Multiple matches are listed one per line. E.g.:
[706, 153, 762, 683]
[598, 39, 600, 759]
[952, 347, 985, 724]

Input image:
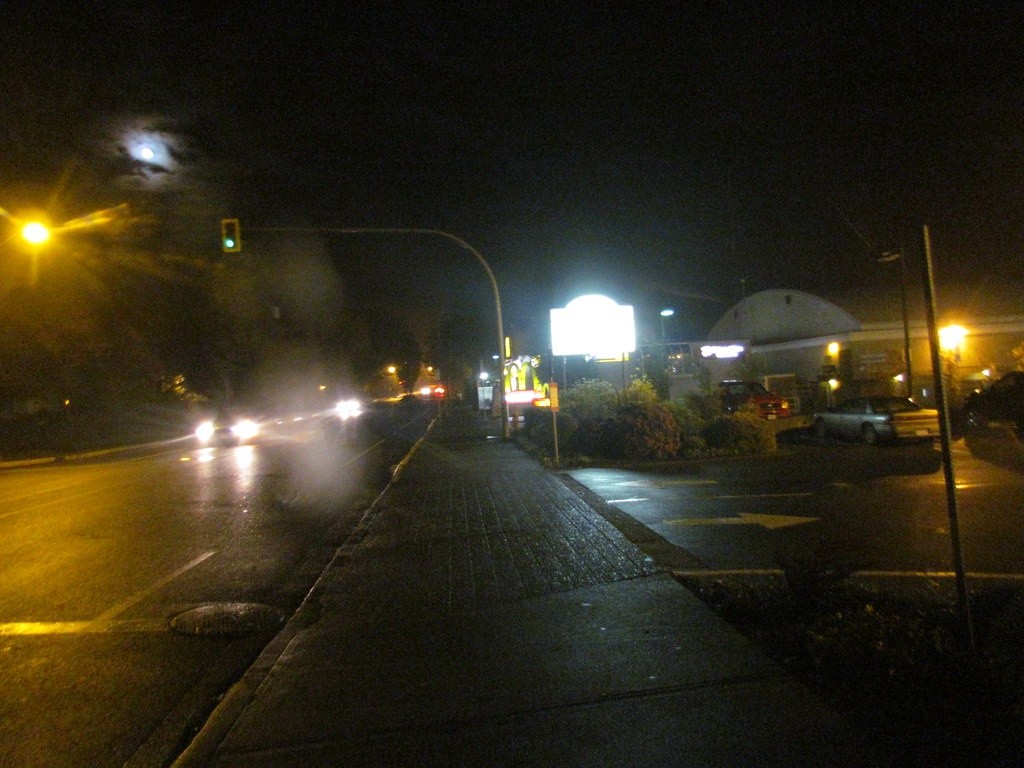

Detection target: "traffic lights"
[220, 218, 242, 253]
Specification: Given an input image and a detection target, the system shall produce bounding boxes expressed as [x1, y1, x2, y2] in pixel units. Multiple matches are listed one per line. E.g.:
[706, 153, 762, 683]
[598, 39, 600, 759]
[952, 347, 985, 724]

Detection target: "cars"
[195, 415, 258, 445]
[718, 379, 794, 420]
[813, 395, 942, 447]
[959, 370, 1024, 437]
